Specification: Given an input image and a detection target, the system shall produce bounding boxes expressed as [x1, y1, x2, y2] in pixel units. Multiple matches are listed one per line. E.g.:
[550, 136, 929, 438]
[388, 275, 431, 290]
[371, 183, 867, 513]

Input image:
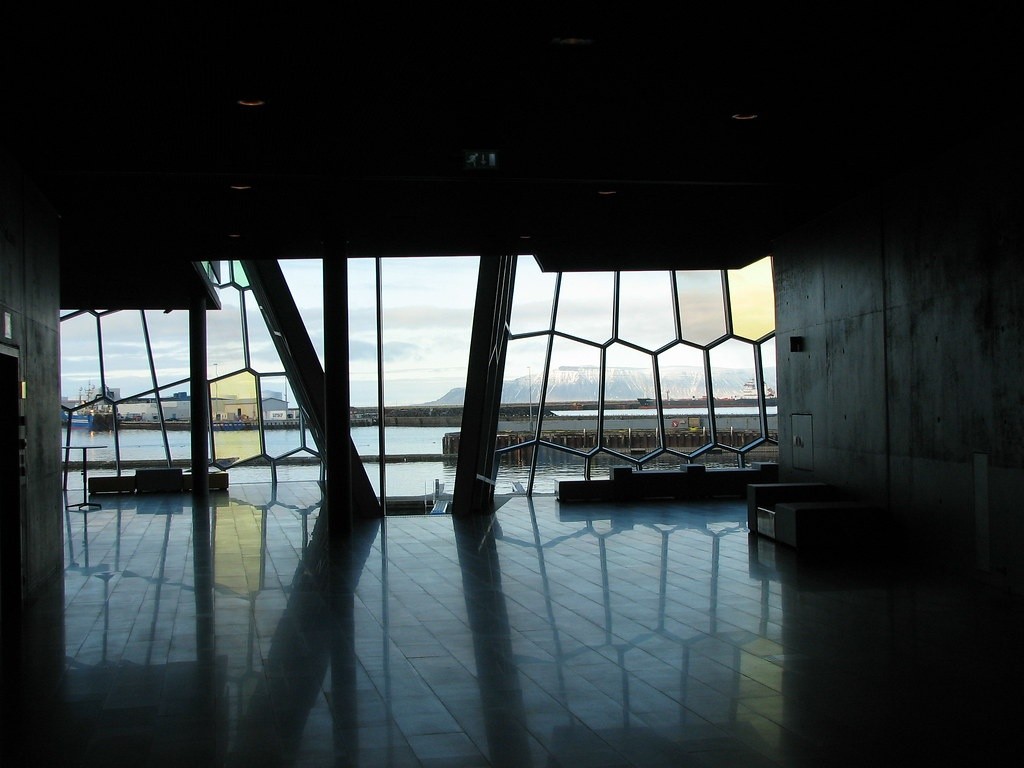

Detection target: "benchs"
[88, 468, 229, 495]
[553, 462, 778, 502]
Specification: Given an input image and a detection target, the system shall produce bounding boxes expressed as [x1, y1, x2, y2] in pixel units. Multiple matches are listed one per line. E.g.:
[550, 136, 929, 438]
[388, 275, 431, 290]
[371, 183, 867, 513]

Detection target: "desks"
[62, 446, 108, 511]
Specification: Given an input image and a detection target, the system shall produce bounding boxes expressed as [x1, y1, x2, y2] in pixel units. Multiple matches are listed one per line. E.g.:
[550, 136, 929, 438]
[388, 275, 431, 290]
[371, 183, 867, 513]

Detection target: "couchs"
[746, 481, 882, 548]
